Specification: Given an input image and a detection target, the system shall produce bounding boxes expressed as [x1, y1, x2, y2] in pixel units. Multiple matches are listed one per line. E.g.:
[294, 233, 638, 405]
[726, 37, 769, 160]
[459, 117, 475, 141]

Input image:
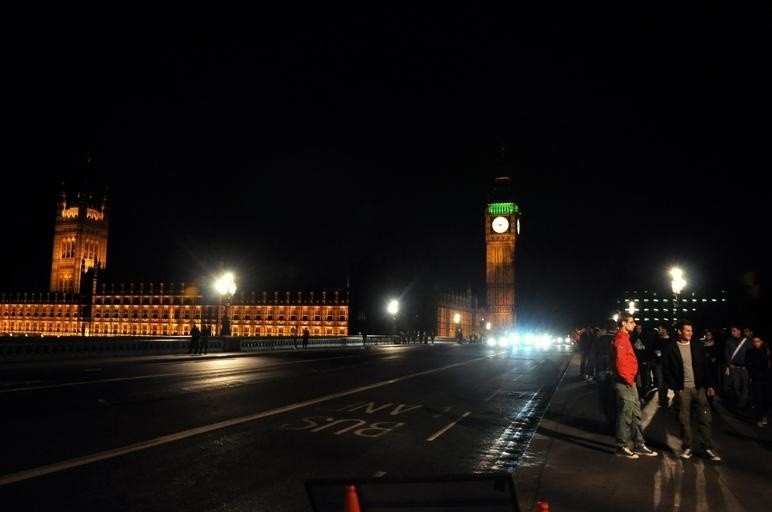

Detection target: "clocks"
[491, 215, 520, 235]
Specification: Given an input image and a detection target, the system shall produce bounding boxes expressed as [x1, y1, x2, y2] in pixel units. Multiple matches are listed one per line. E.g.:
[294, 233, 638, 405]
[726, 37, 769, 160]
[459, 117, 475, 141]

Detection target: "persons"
[199, 325, 210, 355]
[567, 310, 772, 463]
[400, 328, 435, 345]
[188, 323, 202, 354]
[455, 329, 481, 345]
[301, 332, 309, 350]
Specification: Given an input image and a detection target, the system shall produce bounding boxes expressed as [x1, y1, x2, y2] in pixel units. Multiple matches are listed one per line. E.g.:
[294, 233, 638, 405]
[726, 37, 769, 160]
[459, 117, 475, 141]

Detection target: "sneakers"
[705, 448, 720, 461]
[757, 417, 768, 427]
[616, 446, 639, 459]
[679, 448, 692, 459]
[634, 443, 658, 456]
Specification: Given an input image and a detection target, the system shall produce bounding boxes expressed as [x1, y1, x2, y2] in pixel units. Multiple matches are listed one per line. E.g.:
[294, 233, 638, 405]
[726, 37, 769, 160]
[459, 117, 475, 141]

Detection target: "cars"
[468, 330, 577, 350]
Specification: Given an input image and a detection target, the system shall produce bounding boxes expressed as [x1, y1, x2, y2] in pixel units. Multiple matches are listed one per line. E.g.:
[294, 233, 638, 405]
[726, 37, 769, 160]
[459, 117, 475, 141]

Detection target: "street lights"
[212, 272, 239, 337]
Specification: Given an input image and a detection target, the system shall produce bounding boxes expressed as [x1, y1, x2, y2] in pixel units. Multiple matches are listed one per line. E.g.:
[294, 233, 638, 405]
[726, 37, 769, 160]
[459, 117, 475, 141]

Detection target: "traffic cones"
[343, 483, 363, 512]
[533, 497, 551, 511]
